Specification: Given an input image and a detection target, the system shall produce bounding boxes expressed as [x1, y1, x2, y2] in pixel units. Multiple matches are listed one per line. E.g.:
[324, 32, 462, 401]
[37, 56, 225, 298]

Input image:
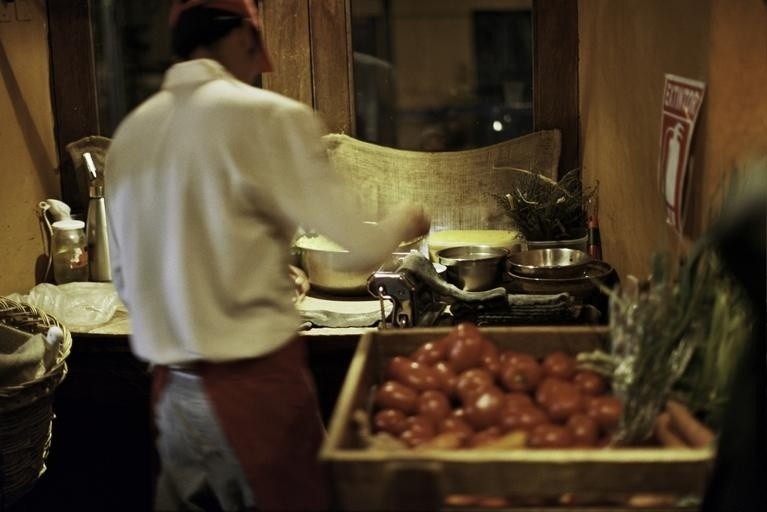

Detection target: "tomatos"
[371, 318, 622, 451]
[447, 491, 677, 511]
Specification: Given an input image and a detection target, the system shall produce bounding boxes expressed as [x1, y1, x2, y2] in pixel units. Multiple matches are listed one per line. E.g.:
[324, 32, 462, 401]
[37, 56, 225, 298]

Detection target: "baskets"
[1, 297, 74, 492]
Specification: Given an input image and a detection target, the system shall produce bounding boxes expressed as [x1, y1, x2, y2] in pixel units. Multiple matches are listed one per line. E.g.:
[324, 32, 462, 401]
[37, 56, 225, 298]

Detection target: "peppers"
[347, 409, 529, 457]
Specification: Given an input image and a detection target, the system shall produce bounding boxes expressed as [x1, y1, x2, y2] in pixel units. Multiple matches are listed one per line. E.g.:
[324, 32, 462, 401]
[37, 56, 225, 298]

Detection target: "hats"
[167, 0, 272, 72]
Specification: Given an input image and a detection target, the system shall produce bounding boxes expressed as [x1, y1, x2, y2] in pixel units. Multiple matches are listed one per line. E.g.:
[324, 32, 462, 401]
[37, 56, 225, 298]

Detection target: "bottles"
[86, 178, 111, 282]
[51, 220, 90, 286]
[588, 215, 602, 260]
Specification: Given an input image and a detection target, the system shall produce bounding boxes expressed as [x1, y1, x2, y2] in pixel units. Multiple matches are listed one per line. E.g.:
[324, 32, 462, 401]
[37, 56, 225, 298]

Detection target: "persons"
[105, 2, 431, 511]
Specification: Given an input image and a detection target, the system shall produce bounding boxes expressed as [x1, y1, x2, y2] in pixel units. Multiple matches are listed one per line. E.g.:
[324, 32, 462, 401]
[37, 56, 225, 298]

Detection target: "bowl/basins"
[433, 245, 509, 294]
[294, 216, 424, 295]
[506, 247, 591, 276]
